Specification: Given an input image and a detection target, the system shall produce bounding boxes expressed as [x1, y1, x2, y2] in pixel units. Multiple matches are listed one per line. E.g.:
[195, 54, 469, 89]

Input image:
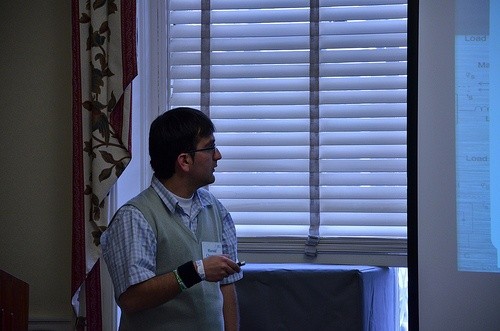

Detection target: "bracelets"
[173, 259, 206, 291]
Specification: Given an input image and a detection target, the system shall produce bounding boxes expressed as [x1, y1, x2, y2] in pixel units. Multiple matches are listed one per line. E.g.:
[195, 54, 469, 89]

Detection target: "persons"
[100, 107, 244, 331]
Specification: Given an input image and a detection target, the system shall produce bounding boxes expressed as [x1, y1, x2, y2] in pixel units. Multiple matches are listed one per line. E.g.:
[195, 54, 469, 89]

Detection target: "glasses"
[187, 144, 218, 153]
